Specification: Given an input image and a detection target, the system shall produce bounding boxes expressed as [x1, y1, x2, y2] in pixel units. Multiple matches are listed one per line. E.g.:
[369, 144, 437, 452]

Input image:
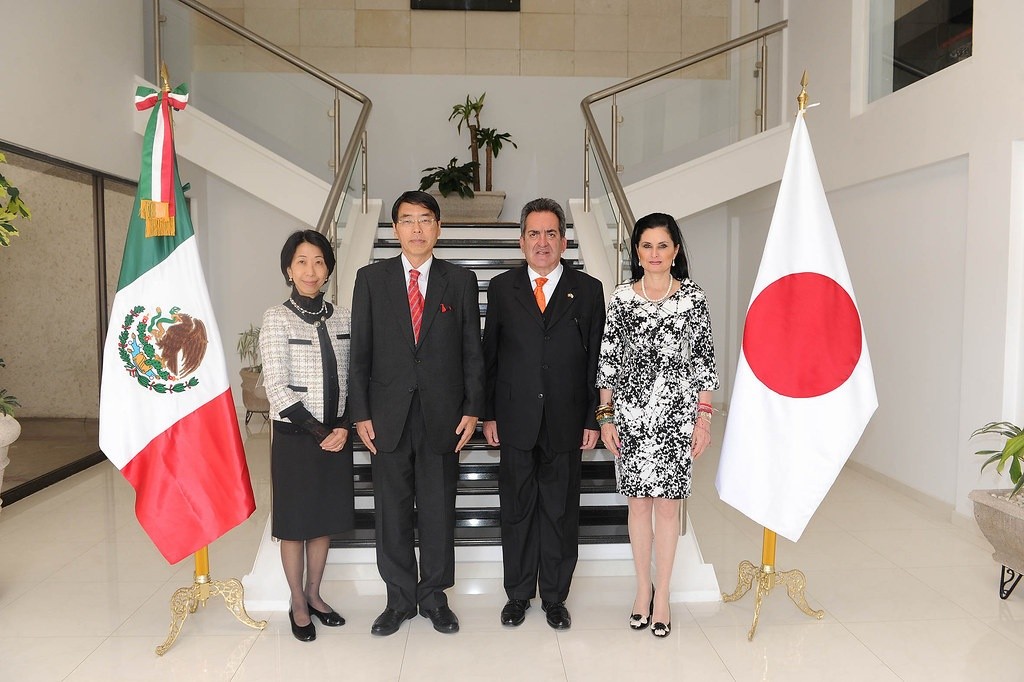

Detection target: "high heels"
[630, 584, 654, 629]
[650, 591, 671, 637]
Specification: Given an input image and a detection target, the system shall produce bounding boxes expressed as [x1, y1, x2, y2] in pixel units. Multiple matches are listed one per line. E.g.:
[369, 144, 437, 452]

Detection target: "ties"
[533, 278, 549, 314]
[408, 270, 425, 347]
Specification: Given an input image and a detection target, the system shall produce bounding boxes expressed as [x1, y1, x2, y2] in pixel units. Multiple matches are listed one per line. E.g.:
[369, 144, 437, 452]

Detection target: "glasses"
[395, 217, 437, 226]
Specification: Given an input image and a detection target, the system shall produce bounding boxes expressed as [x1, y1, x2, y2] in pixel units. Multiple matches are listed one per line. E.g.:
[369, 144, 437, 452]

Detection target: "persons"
[350, 190, 484, 637]
[483, 198, 606, 630]
[258, 228, 351, 642]
[595, 212, 721, 639]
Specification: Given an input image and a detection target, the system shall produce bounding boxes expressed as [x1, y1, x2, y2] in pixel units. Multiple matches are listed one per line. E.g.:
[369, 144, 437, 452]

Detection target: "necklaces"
[287, 297, 330, 316]
[641, 274, 673, 301]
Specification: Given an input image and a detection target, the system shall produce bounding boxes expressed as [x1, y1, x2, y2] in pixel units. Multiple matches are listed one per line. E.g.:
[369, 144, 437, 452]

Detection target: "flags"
[714, 102, 880, 542]
[98, 82, 256, 566]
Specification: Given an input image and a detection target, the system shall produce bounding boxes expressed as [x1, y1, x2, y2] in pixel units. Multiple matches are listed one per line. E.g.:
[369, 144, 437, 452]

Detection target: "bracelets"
[696, 402, 720, 424]
[595, 402, 615, 427]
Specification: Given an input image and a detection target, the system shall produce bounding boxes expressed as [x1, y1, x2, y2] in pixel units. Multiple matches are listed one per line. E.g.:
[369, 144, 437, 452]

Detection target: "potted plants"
[969, 421, 1024, 578]
[421, 91, 517, 223]
[0, 153, 32, 489]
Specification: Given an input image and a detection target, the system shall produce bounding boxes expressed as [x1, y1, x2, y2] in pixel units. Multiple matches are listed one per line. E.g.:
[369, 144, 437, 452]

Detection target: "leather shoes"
[542, 599, 571, 629]
[307, 601, 345, 626]
[419, 592, 460, 633]
[372, 606, 417, 636]
[289, 606, 316, 641]
[501, 599, 530, 627]
